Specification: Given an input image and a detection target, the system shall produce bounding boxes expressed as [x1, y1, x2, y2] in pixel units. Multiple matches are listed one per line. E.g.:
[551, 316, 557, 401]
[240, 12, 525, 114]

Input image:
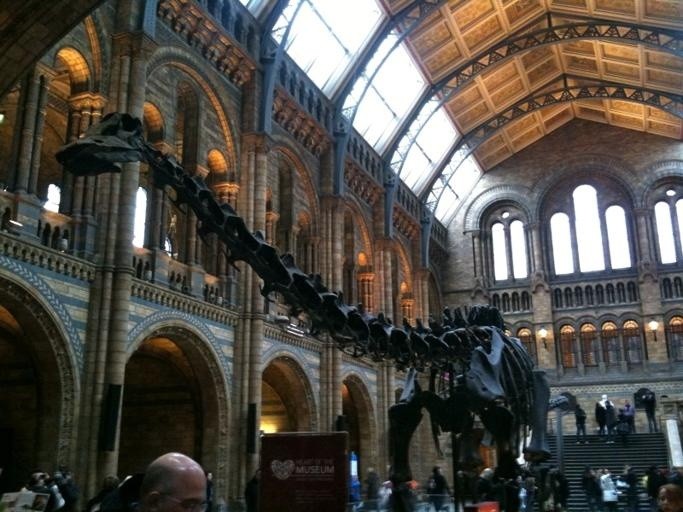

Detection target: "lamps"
[538, 327, 549, 349]
[648, 318, 661, 342]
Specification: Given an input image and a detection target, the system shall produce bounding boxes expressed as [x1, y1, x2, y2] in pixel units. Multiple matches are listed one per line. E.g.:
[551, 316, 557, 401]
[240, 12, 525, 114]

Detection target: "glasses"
[162, 495, 208, 511]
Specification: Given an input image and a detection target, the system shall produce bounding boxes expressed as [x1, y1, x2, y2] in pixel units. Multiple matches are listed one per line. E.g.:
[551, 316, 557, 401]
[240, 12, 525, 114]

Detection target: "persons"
[351, 464, 570, 512]
[624, 403, 637, 434]
[574, 403, 590, 445]
[19, 452, 214, 511]
[604, 408, 616, 445]
[241, 469, 259, 512]
[641, 389, 659, 433]
[595, 402, 607, 435]
[617, 408, 631, 446]
[606, 401, 616, 435]
[581, 461, 683, 512]
